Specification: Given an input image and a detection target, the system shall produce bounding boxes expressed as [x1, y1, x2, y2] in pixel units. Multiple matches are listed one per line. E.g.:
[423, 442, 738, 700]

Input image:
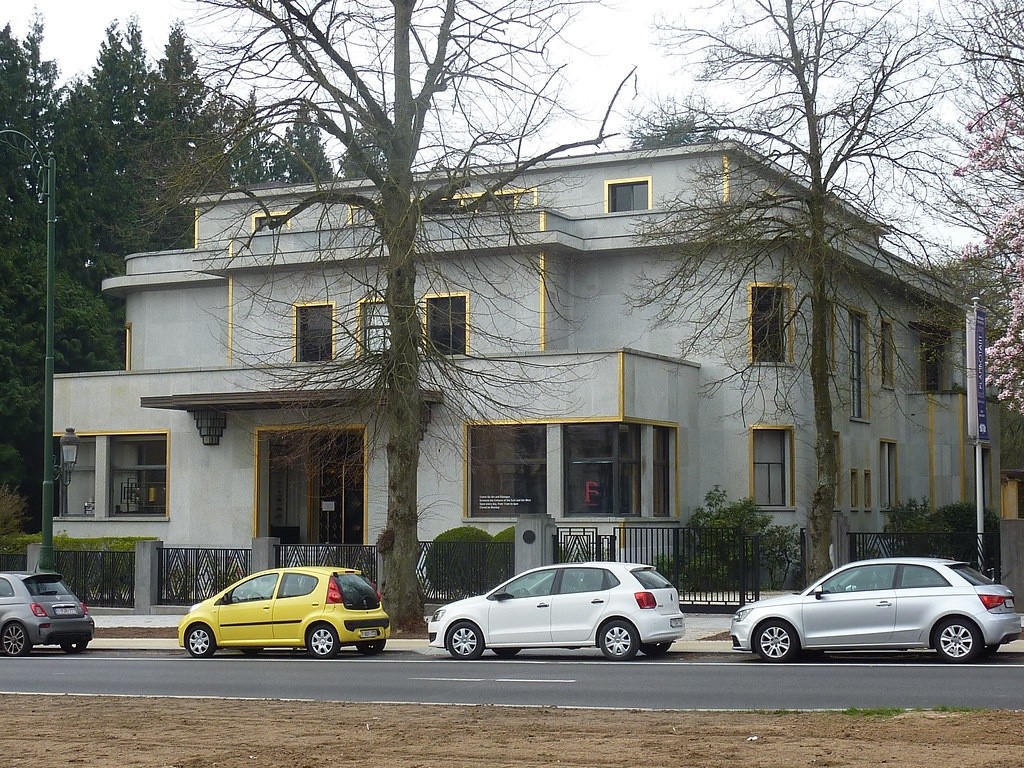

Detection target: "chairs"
[285, 576, 313, 596]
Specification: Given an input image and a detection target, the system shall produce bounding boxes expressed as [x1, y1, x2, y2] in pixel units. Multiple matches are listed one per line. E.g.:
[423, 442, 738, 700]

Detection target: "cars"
[176, 566, 390, 659]
[0, 567, 95, 659]
[729, 556, 1022, 665]
[427, 561, 687, 659]
